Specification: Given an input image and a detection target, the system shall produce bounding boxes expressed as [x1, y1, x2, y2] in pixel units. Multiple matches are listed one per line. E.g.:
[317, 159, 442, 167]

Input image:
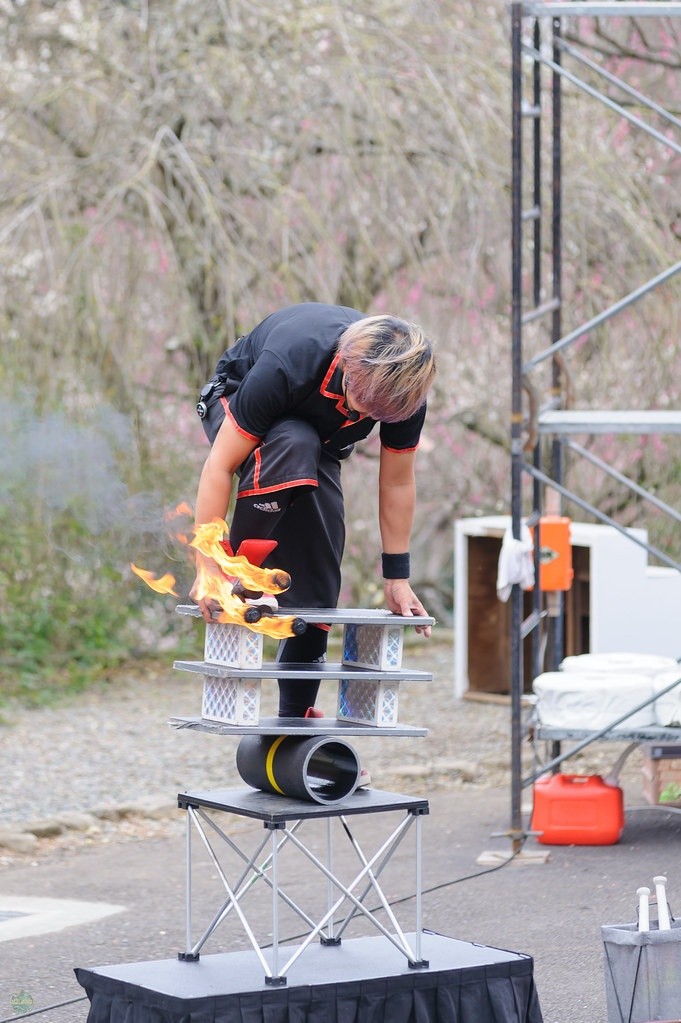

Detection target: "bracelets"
[380, 551, 410, 580]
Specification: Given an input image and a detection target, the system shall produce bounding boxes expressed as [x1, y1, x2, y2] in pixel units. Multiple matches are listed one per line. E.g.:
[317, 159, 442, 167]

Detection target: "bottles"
[532, 774, 623, 845]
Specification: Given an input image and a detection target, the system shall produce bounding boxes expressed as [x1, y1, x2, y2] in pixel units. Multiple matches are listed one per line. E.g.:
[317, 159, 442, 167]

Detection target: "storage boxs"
[532, 774, 626, 846]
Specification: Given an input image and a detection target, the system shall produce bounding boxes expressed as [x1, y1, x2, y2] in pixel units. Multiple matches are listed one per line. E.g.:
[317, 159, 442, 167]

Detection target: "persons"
[195, 303, 439, 791]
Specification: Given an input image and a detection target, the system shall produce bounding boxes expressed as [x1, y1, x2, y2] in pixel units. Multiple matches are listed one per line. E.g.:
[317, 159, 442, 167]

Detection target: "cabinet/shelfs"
[512, 0, 680, 850]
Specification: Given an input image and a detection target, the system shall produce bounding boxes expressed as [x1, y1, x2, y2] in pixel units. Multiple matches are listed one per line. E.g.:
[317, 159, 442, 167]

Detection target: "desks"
[73, 928, 545, 1023]
[177, 786, 429, 986]
